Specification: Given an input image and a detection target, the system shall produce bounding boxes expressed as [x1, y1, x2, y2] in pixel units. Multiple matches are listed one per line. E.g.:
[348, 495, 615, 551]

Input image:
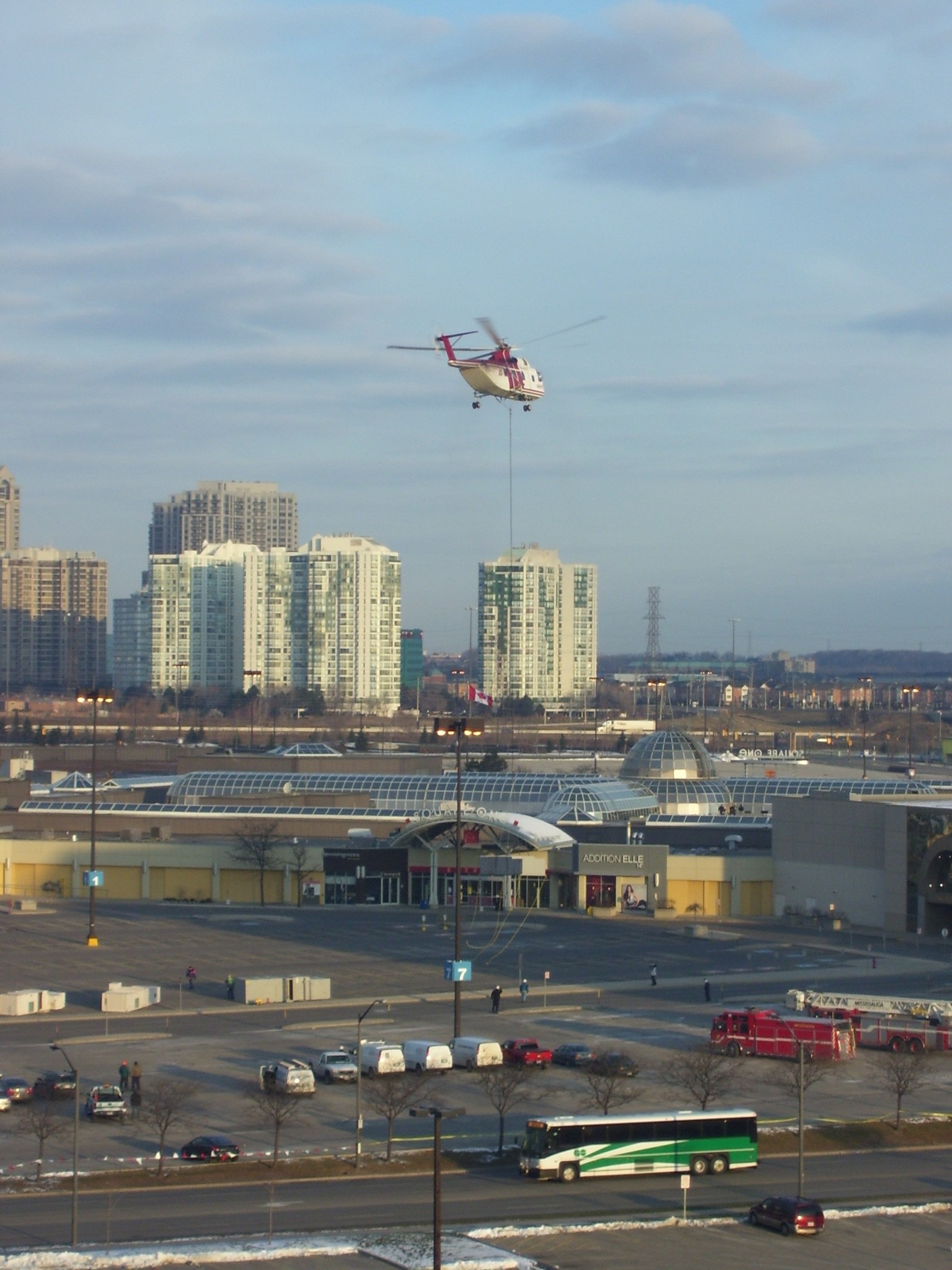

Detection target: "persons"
[119, 1061, 141, 1090]
[624, 885, 646, 909]
[650, 964, 656, 985]
[131, 1089, 141, 1120]
[520, 979, 528, 1002]
[491, 985, 502, 1013]
[718, 803, 744, 816]
[225, 975, 233, 999]
[704, 978, 710, 1001]
[186, 966, 196, 989]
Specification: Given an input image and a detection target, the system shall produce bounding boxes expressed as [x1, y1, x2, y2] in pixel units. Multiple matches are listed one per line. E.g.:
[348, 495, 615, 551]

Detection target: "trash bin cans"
[493, 897, 503, 910]
[420, 900, 428, 908]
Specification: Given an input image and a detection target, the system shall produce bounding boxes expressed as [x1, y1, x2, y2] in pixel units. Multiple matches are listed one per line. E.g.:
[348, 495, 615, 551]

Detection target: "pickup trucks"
[86, 1084, 128, 1120]
[500, 1037, 552, 1071]
[313, 1051, 359, 1084]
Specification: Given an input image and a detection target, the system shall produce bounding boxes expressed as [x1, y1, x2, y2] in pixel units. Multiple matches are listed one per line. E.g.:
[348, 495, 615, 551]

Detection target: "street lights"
[172, 664, 189, 747]
[451, 670, 465, 716]
[243, 670, 262, 745]
[729, 618, 740, 754]
[647, 678, 667, 731]
[409, 1105, 467, 1270]
[48, 1041, 80, 1246]
[699, 670, 713, 747]
[767, 1012, 805, 1197]
[589, 677, 604, 772]
[858, 676, 872, 779]
[354, 998, 390, 1173]
[76, 689, 114, 946]
[433, 717, 484, 1035]
[902, 685, 919, 779]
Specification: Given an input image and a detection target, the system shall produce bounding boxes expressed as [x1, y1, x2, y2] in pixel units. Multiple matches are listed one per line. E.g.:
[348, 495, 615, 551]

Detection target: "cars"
[180, 1134, 240, 1164]
[0, 1074, 34, 1103]
[551, 1043, 596, 1069]
[33, 1070, 76, 1101]
[595, 1052, 639, 1078]
[0, 1086, 11, 1111]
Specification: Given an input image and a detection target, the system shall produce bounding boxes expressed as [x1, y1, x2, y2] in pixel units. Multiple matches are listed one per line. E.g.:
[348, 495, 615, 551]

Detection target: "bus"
[514, 1107, 760, 1183]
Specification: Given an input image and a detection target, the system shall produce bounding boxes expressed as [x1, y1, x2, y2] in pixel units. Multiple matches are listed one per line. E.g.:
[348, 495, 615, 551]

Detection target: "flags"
[469, 685, 493, 706]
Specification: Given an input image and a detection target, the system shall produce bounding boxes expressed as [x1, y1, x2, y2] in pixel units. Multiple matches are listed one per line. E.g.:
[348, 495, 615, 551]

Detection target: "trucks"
[259, 1057, 316, 1099]
[348, 1038, 406, 1082]
[445, 1035, 503, 1073]
[400, 1040, 453, 1076]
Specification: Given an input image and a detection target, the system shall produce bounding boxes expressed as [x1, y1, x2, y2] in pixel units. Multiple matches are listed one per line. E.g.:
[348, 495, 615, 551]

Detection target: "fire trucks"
[710, 1006, 856, 1064]
[783, 988, 952, 1055]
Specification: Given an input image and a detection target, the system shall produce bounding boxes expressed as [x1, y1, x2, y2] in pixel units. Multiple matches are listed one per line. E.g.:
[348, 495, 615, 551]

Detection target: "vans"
[749, 1195, 825, 1237]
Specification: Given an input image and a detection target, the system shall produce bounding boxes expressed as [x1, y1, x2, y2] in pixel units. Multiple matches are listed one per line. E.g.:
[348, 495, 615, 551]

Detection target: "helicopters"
[386, 314, 603, 413]
